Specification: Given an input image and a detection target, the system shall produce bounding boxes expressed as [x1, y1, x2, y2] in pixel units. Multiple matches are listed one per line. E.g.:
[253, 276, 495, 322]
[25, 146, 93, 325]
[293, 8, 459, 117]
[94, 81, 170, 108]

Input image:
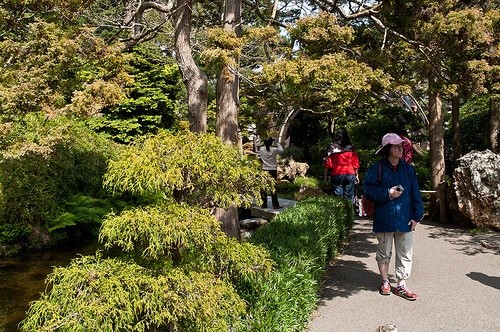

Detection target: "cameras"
[397, 185, 404, 192]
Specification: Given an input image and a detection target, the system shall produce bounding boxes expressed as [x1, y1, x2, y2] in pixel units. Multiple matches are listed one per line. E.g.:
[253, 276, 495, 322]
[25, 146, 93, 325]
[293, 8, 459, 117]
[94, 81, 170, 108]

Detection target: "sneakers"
[392, 284, 417, 301]
[380, 278, 393, 295]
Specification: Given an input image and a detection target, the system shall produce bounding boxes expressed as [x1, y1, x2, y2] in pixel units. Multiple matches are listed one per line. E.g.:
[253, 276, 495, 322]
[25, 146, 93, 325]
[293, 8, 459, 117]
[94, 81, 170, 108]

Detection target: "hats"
[374, 133, 407, 155]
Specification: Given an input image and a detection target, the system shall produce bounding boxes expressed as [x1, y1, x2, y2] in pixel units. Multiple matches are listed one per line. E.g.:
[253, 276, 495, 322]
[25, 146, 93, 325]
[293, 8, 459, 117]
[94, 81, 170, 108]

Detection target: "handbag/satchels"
[357, 159, 382, 218]
[354, 179, 367, 217]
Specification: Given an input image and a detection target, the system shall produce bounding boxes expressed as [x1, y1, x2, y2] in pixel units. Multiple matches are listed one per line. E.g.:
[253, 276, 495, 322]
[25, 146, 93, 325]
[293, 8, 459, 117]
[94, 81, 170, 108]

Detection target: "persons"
[255, 137, 285, 209]
[361, 132, 423, 300]
[399, 129, 413, 163]
[323, 143, 361, 214]
